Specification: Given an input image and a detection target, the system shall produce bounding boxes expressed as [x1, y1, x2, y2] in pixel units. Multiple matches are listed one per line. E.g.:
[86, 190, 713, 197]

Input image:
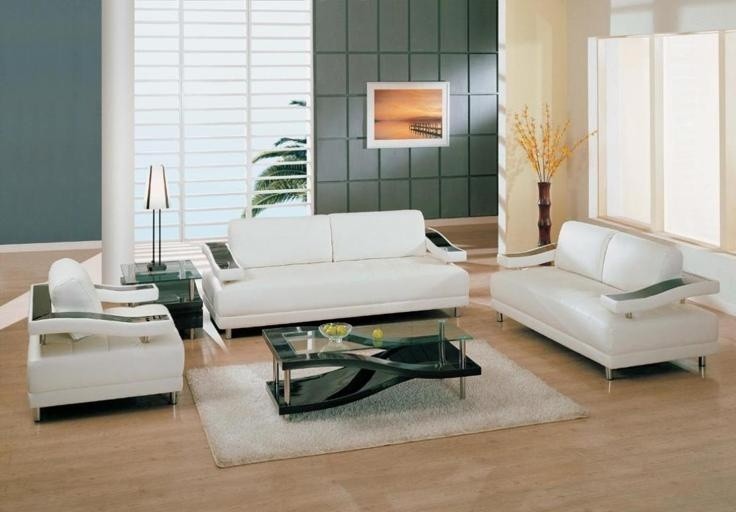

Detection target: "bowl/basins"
[319, 322, 352, 341]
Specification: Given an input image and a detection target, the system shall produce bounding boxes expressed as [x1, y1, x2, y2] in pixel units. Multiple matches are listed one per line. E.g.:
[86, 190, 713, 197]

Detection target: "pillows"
[603, 231, 684, 292]
[230, 215, 332, 268]
[554, 220, 617, 284]
[329, 210, 426, 261]
[50, 259, 103, 340]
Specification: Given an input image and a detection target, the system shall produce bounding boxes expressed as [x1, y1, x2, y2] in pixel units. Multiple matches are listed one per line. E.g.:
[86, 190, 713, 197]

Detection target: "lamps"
[143, 163, 170, 271]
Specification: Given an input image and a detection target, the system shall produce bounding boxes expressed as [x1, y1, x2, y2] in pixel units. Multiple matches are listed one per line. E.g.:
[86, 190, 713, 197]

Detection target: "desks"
[120, 260, 203, 339]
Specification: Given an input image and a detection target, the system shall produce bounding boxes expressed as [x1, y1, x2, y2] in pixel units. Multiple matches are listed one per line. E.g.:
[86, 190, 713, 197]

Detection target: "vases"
[538, 182, 552, 246]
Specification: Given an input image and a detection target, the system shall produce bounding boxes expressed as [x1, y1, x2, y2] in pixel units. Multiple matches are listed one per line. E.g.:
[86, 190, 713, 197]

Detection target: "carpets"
[185, 343, 591, 471]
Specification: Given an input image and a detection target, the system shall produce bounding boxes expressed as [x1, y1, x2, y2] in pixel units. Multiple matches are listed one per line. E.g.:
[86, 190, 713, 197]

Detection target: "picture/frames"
[366, 81, 451, 149]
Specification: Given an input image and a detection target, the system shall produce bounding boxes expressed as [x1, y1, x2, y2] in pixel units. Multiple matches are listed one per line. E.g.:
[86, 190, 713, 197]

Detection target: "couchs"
[201, 210, 469, 341]
[26, 282, 185, 424]
[489, 222, 720, 381]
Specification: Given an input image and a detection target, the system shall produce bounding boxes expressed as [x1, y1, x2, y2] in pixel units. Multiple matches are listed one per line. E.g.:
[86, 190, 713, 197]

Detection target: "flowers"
[512, 101, 598, 183]
